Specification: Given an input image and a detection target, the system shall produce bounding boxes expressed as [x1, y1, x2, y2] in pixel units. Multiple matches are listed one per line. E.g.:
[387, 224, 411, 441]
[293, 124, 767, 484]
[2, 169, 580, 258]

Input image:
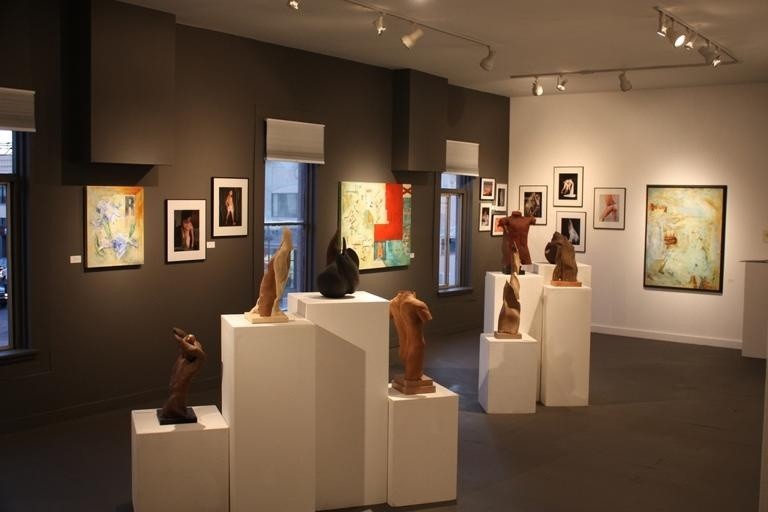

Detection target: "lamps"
[480, 46, 496, 71]
[657, 13, 722, 69]
[288, 0, 299, 10]
[400, 23, 424, 49]
[556, 76, 568, 91]
[619, 71, 633, 91]
[532, 77, 543, 96]
[373, 13, 385, 34]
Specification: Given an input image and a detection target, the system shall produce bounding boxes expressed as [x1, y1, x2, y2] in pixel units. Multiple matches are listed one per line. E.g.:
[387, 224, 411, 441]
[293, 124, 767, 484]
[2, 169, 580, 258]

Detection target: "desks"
[541, 284, 593, 408]
[220, 312, 319, 512]
[131, 403, 230, 511]
[484, 270, 545, 402]
[533, 261, 593, 286]
[522, 264, 533, 272]
[286, 289, 392, 511]
[387, 380, 461, 508]
[476, 330, 540, 416]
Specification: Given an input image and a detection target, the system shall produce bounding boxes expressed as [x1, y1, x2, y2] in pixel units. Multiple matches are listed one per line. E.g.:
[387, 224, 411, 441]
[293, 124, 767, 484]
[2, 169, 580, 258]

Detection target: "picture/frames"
[162, 197, 207, 266]
[644, 184, 727, 295]
[82, 184, 147, 272]
[519, 185, 548, 225]
[553, 166, 584, 207]
[209, 175, 250, 240]
[593, 187, 626, 230]
[478, 178, 508, 237]
[556, 211, 587, 252]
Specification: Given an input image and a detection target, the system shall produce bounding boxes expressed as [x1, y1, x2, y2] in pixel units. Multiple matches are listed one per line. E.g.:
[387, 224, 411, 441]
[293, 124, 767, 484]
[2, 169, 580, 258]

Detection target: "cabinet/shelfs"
[741, 262, 768, 359]
